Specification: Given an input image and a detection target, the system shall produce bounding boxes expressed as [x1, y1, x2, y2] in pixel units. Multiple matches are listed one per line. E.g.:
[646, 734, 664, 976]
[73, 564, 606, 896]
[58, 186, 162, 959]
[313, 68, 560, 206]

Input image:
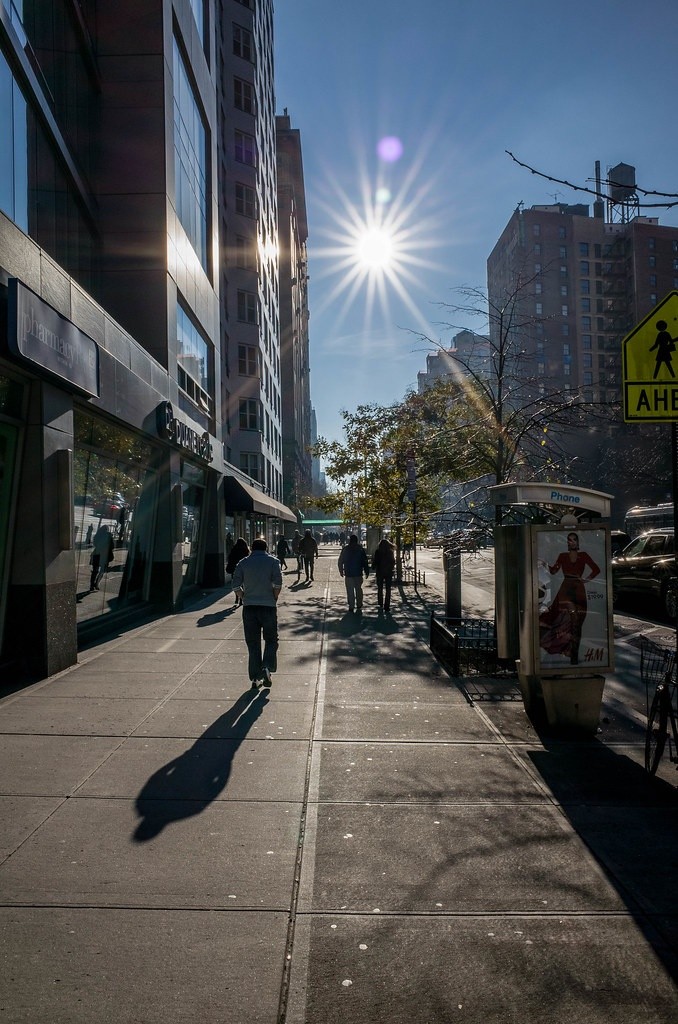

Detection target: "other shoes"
[357, 604, 363, 609]
[310, 575, 314, 581]
[251, 679, 263, 690]
[262, 668, 272, 687]
[306, 576, 309, 580]
[380, 604, 383, 608]
[349, 609, 353, 612]
[384, 607, 389, 612]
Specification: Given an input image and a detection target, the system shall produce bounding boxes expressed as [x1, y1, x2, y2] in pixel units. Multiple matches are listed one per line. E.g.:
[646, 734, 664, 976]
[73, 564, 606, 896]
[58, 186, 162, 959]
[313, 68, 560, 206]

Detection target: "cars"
[95, 491, 131, 510]
[609, 526, 677, 625]
[360, 525, 494, 551]
[610, 530, 630, 556]
[92, 498, 129, 519]
[75, 494, 93, 506]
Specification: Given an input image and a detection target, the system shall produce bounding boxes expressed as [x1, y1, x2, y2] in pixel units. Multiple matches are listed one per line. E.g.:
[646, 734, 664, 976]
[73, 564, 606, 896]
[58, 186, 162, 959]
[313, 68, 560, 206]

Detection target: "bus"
[624, 501, 676, 542]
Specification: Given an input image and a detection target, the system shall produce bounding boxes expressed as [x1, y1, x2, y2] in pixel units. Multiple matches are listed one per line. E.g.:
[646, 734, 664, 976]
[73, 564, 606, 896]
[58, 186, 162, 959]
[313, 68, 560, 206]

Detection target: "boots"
[235, 595, 242, 606]
[90, 572, 103, 591]
[570, 624, 581, 665]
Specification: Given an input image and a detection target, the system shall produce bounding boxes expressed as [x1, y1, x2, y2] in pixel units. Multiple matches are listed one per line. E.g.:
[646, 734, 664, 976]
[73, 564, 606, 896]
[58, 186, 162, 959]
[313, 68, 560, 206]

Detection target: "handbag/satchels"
[108, 550, 114, 562]
[226, 559, 237, 574]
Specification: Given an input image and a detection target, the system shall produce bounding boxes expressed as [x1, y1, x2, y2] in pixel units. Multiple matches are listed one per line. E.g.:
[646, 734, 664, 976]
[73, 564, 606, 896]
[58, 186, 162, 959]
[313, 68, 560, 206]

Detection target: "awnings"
[224, 476, 297, 524]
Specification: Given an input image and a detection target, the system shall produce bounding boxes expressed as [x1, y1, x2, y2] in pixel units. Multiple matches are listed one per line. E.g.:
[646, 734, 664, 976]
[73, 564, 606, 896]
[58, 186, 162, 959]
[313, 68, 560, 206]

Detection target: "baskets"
[641, 642, 678, 685]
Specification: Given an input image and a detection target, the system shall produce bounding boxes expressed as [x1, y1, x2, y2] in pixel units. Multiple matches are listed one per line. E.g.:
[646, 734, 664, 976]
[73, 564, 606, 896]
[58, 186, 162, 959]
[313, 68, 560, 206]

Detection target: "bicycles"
[108, 519, 131, 541]
[636, 632, 678, 776]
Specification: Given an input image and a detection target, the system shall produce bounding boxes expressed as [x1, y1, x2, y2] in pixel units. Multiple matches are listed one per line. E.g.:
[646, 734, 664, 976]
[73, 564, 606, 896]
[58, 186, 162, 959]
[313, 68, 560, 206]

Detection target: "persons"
[89, 525, 114, 591]
[338, 535, 369, 612]
[277, 529, 319, 582]
[227, 538, 249, 605]
[371, 540, 395, 610]
[232, 539, 282, 688]
[537, 532, 600, 665]
[314, 530, 345, 546]
